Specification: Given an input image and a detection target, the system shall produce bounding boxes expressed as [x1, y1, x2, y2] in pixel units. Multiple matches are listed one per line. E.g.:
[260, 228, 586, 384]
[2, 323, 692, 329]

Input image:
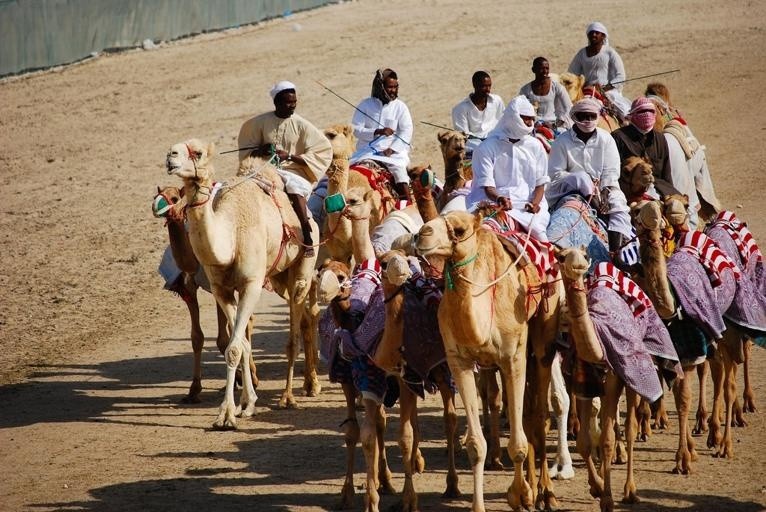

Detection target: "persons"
[237, 80, 334, 258]
[518, 57, 575, 133]
[645, 82, 682, 127]
[451, 71, 505, 161]
[566, 21, 631, 125]
[349, 67, 413, 202]
[546, 98, 633, 271]
[465, 95, 550, 243]
[610, 97, 683, 199]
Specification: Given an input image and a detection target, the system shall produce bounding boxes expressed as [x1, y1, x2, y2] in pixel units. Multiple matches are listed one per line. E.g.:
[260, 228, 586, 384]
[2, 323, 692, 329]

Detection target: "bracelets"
[496, 194, 503, 204]
[605, 187, 612, 193]
[288, 153, 292, 162]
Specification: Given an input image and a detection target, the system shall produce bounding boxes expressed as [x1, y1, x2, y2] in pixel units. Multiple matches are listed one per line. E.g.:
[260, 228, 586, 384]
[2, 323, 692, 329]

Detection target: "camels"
[315, 67, 766, 512]
